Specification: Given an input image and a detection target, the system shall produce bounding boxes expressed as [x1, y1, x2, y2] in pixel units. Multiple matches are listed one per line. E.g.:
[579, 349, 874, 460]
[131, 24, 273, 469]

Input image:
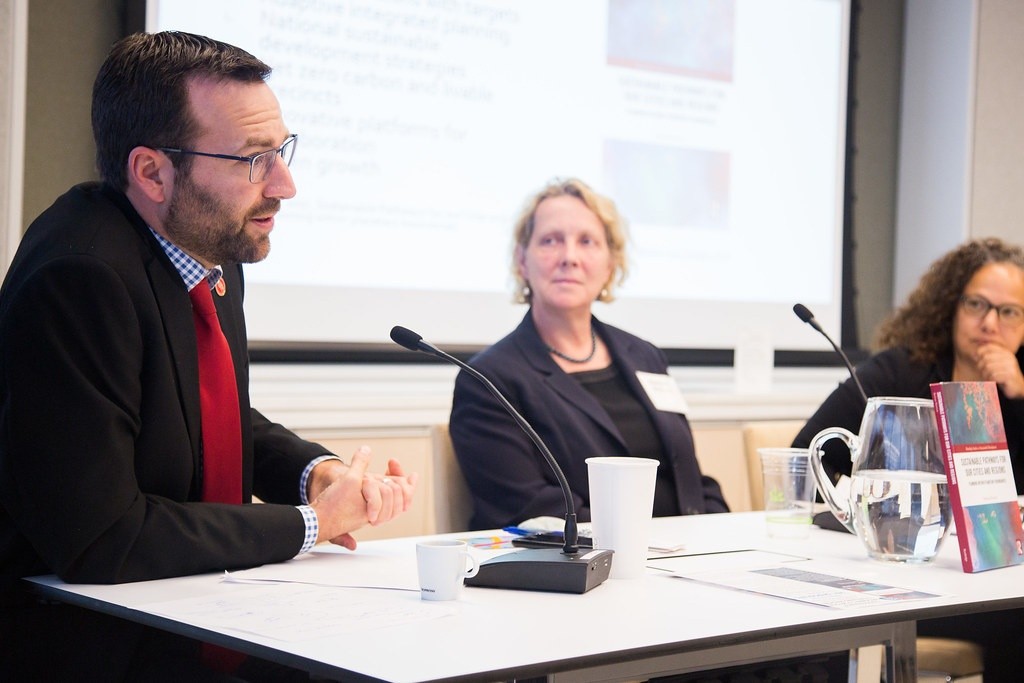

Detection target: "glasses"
[958, 294, 1024, 328]
[155, 134, 298, 183]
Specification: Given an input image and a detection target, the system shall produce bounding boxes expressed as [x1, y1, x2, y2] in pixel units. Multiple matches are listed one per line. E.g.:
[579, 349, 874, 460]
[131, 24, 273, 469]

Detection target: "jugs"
[807, 397, 953, 563]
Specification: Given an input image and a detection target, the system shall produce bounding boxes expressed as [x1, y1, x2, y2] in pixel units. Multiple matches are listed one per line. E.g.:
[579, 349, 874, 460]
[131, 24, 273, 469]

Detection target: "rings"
[382, 478, 391, 484]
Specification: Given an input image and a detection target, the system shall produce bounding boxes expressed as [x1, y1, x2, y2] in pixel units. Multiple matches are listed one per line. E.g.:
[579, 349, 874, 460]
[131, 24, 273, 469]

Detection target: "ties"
[188, 277, 249, 674]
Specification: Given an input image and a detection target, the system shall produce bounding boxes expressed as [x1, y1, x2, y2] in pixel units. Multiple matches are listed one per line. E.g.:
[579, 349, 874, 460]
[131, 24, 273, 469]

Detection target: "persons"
[789, 236, 1024, 683]
[449, 179, 731, 531]
[0, 31, 417, 683]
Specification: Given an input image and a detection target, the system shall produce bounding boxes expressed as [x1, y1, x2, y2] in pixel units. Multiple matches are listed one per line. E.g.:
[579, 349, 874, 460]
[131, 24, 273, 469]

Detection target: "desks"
[18, 497, 1024, 683]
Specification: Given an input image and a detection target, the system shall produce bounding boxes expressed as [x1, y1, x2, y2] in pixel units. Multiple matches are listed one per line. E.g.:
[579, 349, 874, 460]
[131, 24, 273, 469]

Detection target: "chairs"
[745, 421, 979, 676]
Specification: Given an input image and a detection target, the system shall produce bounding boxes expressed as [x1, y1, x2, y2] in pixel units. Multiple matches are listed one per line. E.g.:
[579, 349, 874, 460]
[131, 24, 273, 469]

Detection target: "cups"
[757, 448, 825, 542]
[416, 540, 479, 601]
[584, 456, 660, 578]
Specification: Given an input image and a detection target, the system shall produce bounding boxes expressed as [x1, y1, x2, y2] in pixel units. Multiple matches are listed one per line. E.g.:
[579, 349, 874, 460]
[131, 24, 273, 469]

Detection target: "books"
[929, 381, 1024, 572]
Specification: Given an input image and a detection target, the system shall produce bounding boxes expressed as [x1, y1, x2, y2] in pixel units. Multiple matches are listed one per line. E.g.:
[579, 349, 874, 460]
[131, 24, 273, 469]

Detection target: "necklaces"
[545, 329, 597, 364]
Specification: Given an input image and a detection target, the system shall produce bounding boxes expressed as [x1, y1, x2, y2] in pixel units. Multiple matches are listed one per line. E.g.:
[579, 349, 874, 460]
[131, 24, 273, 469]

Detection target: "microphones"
[390, 323, 614, 594]
[792, 303, 870, 533]
